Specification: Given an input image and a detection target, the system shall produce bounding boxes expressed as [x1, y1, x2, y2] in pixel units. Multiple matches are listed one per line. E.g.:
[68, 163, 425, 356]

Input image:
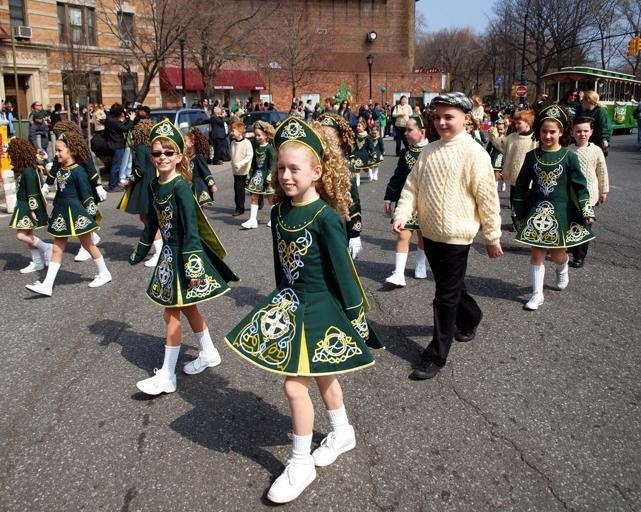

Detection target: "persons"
[0, 97, 216, 297]
[128, 120, 240, 395]
[425, 91, 639, 311]
[391, 91, 506, 380]
[225, 115, 388, 506]
[212, 95, 432, 286]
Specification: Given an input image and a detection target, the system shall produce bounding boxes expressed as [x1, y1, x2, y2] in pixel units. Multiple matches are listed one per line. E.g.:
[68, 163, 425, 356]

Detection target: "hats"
[431, 92, 472, 111]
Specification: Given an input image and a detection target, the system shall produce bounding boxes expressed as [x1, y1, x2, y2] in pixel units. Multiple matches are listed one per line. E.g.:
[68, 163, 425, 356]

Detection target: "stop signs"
[515, 85, 528, 98]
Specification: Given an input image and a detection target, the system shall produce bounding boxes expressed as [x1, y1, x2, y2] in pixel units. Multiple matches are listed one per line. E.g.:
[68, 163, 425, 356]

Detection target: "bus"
[539, 66, 641, 134]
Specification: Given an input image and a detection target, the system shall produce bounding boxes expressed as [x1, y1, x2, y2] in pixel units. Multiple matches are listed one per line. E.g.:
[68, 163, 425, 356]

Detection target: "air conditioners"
[13, 25, 32, 39]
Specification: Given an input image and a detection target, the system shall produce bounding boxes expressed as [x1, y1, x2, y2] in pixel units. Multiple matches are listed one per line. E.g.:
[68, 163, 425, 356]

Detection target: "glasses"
[152, 151, 174, 157]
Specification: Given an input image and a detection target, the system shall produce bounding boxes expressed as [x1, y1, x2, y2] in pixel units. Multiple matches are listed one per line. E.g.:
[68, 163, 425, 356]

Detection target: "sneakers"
[525, 292, 545, 310]
[145, 254, 160, 267]
[415, 263, 427, 279]
[385, 271, 406, 288]
[267, 426, 356, 504]
[241, 219, 258, 228]
[456, 311, 482, 342]
[233, 208, 244, 216]
[19, 232, 112, 297]
[136, 368, 177, 395]
[183, 348, 222, 374]
[414, 362, 439, 379]
[556, 255, 583, 289]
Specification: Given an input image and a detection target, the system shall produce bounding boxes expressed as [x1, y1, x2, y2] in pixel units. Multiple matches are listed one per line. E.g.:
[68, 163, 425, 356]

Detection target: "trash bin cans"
[11, 119, 30, 141]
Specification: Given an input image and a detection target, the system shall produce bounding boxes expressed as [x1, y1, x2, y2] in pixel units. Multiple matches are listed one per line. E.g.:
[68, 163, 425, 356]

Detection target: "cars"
[241, 110, 289, 147]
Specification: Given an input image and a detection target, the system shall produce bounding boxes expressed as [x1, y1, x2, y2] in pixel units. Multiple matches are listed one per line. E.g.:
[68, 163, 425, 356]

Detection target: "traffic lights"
[635, 35, 640, 52]
[627, 38, 635, 56]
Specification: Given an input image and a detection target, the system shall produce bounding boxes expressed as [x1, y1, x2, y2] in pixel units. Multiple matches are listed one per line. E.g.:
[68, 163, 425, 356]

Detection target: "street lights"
[175, 24, 189, 106]
[363, 52, 374, 102]
[420, 86, 426, 105]
[380, 84, 385, 107]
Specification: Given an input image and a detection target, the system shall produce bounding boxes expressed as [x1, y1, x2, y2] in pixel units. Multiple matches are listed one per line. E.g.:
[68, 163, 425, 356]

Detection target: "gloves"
[348, 236, 362, 260]
[42, 183, 51, 196]
[95, 185, 107, 201]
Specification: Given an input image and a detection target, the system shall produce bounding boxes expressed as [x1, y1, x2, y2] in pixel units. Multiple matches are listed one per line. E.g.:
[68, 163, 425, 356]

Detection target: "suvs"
[143, 106, 212, 148]
